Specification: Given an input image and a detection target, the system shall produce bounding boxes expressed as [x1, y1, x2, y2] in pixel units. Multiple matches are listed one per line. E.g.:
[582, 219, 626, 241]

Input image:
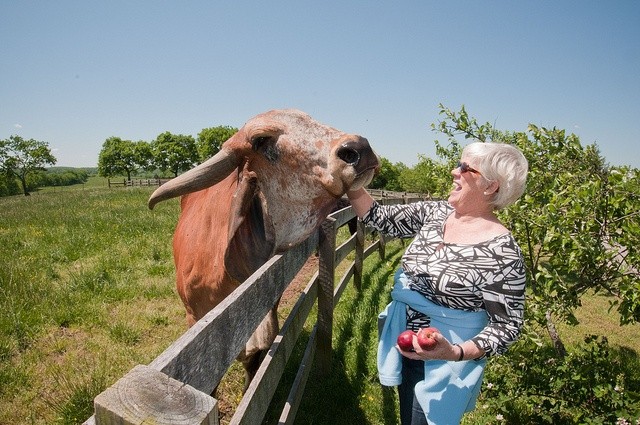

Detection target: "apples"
[398, 330, 417, 351]
[418, 328, 438, 352]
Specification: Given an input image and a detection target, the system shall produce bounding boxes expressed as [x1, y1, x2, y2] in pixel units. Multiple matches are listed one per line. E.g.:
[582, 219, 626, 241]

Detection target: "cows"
[147, 107, 384, 397]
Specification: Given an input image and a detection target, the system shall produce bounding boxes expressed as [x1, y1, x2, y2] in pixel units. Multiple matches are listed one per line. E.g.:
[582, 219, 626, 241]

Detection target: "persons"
[345, 140, 532, 424]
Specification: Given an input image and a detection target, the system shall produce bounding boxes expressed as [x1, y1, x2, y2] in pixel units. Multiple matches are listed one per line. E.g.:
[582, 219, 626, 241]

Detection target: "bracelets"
[453, 342, 464, 362]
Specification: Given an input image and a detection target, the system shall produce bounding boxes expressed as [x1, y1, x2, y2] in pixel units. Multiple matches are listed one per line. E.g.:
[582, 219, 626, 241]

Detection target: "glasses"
[456, 160, 480, 177]
